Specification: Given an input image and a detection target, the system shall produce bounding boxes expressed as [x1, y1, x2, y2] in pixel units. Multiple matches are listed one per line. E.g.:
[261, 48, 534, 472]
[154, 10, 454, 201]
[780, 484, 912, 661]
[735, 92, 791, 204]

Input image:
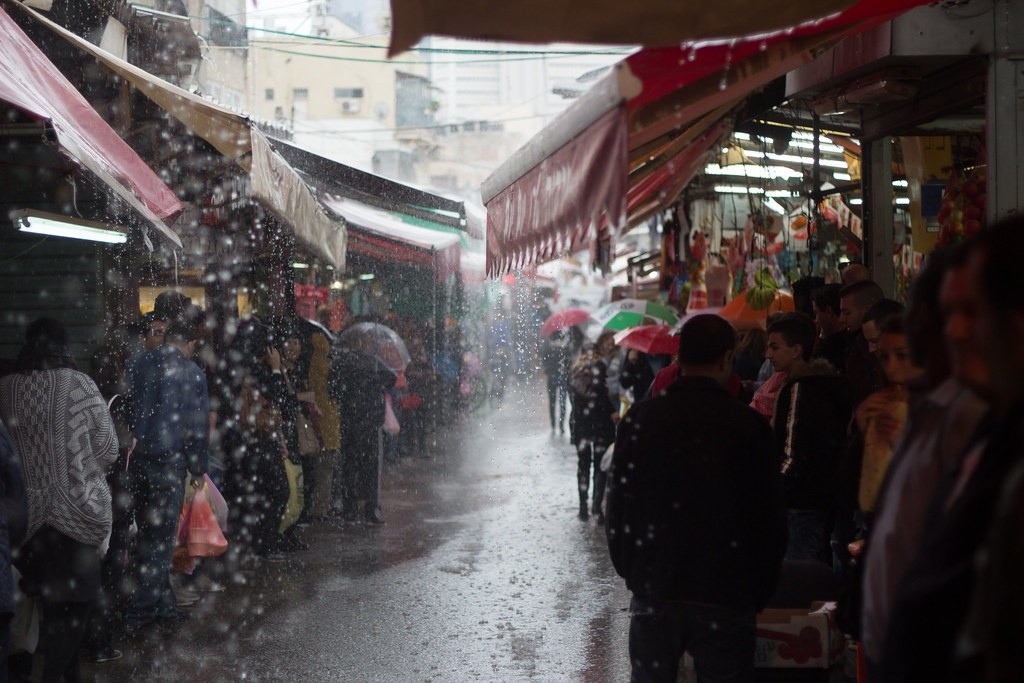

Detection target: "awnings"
[1, 0, 485, 277]
[387, 1, 936, 281]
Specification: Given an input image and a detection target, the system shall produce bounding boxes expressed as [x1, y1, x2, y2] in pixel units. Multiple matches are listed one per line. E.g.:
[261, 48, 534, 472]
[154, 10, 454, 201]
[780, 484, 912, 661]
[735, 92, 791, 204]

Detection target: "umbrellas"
[611, 324, 682, 366]
[592, 298, 677, 331]
[719, 288, 794, 328]
[542, 309, 589, 335]
[336, 319, 410, 371]
[668, 307, 723, 336]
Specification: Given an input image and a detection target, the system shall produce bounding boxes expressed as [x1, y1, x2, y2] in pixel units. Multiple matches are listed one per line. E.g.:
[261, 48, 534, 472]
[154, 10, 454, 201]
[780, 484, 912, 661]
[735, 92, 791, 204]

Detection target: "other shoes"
[175, 590, 200, 606]
[343, 513, 362, 526]
[363, 511, 385, 526]
[278, 529, 309, 552]
[580, 502, 604, 523]
[99, 650, 122, 662]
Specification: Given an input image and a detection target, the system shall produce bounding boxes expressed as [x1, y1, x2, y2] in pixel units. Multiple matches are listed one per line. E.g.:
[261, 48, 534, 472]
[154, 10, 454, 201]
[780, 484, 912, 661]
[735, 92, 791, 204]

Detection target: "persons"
[0, 290, 515, 682]
[542, 213, 1024, 682]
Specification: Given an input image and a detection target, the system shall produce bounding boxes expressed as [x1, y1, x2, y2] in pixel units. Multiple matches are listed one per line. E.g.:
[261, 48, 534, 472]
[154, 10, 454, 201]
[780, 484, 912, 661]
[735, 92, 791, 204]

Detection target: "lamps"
[8, 133, 130, 243]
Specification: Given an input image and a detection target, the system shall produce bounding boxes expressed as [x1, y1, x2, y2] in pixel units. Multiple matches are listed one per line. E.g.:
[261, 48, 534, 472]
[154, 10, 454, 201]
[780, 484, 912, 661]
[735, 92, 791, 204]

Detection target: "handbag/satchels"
[296, 412, 320, 457]
[175, 471, 228, 575]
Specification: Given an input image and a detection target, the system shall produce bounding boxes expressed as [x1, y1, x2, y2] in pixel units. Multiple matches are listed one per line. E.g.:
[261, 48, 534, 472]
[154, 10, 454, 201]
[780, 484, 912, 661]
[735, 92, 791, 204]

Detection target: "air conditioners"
[340, 99, 358, 112]
